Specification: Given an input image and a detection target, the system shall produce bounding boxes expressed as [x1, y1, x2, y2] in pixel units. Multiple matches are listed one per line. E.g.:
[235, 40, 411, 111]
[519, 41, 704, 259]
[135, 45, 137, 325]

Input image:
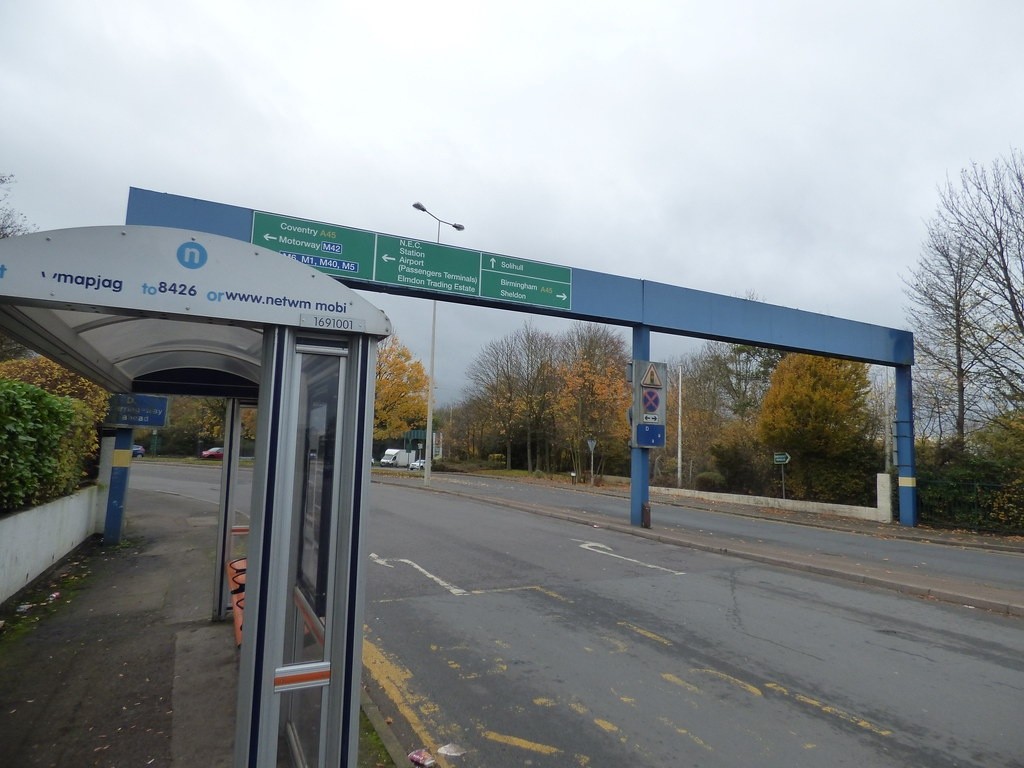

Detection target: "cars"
[202, 447, 224, 461]
[410, 460, 425, 470]
[132, 444, 145, 458]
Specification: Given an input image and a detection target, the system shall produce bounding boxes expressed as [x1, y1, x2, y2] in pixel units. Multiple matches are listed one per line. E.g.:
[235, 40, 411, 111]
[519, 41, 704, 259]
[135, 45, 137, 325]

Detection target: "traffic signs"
[480, 251, 572, 310]
[250, 210, 377, 282]
[375, 233, 480, 295]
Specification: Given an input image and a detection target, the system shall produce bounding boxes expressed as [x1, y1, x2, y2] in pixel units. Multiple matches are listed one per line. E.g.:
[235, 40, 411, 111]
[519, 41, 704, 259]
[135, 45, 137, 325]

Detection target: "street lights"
[413, 202, 466, 487]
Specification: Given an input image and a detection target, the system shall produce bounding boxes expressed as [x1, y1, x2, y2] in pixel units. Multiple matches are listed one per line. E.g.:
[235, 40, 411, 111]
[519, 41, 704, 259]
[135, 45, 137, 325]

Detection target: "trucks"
[380, 449, 415, 468]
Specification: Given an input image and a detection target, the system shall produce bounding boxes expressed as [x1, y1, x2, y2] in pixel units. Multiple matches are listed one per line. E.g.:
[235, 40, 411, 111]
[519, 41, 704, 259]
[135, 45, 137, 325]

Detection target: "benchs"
[227, 558, 317, 651]
